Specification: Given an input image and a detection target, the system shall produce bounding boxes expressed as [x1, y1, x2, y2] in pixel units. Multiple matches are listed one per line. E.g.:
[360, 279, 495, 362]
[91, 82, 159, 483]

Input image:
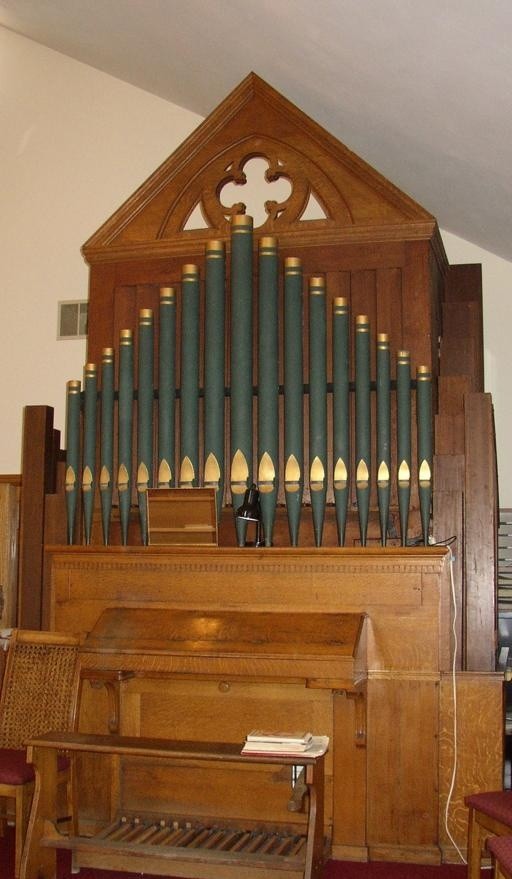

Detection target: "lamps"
[234, 482, 264, 546]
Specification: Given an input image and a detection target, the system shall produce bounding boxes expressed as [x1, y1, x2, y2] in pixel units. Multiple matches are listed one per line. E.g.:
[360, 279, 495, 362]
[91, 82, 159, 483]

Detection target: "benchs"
[17, 728, 328, 878]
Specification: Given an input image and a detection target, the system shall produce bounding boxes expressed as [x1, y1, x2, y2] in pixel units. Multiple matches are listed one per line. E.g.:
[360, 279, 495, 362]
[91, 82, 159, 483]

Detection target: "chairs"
[0, 626, 89, 878]
[463, 784, 512, 879]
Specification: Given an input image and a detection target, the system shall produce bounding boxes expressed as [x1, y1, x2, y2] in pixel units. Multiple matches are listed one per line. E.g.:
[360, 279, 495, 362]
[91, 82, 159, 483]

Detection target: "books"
[240, 728, 329, 758]
